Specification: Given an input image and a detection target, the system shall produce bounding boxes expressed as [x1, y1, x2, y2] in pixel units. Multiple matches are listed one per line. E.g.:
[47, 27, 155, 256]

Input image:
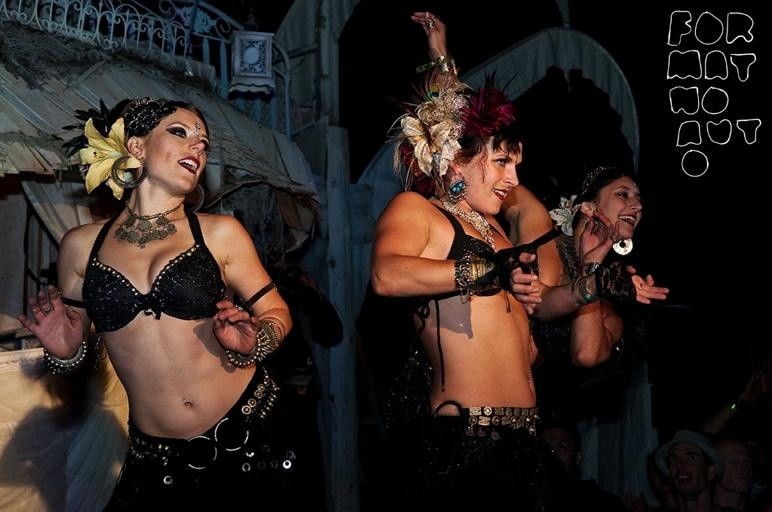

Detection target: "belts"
[432, 400, 540, 442]
[126, 370, 280, 472]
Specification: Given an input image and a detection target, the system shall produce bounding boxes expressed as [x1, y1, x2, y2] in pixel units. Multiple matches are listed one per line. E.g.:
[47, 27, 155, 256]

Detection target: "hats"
[654, 430, 725, 481]
